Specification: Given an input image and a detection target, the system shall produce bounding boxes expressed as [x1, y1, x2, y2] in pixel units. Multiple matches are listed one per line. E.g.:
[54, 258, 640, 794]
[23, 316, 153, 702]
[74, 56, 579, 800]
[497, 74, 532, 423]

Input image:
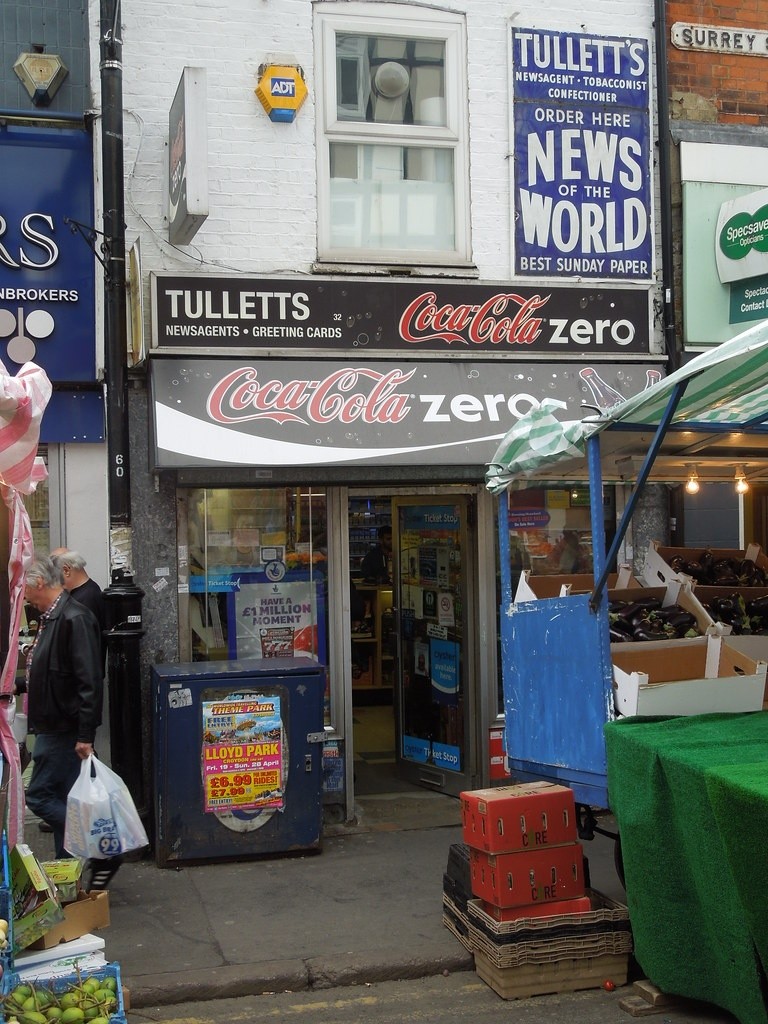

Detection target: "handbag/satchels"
[64, 753, 149, 859]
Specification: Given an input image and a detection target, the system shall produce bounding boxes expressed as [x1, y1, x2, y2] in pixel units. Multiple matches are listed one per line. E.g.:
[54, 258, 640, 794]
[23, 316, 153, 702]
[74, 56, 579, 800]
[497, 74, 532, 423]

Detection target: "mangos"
[6, 977, 115, 1024]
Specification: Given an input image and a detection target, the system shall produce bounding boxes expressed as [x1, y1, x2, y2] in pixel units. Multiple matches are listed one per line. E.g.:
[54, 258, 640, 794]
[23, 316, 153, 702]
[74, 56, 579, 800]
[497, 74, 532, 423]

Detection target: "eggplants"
[609, 597, 699, 644]
[670, 548, 767, 587]
[701, 590, 768, 635]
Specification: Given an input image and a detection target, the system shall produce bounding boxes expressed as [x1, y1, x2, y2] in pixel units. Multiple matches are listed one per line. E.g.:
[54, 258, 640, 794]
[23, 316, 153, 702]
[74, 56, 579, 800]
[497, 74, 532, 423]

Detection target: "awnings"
[484, 318, 768, 496]
[142, 355, 671, 470]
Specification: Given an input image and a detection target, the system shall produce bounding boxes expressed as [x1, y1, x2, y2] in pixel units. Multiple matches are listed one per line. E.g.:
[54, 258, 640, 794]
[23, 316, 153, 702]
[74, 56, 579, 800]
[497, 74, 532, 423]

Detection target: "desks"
[605, 711, 768, 1024]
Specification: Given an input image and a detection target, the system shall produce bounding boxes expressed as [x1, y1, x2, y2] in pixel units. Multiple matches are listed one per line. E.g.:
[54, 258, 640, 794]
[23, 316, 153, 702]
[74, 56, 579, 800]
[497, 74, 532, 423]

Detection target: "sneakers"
[88, 854, 123, 894]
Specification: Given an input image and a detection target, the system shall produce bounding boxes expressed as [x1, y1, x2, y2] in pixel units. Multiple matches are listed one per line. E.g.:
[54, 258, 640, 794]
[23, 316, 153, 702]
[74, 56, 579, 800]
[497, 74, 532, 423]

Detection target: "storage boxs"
[0, 828, 10, 888]
[0, 960, 128, 1024]
[440, 873, 470, 926]
[468, 842, 585, 908]
[441, 902, 474, 953]
[39, 856, 85, 883]
[26, 889, 111, 949]
[459, 779, 578, 855]
[64, 796, 118, 858]
[445, 843, 472, 891]
[509, 541, 768, 717]
[55, 873, 84, 902]
[0, 888, 15, 971]
[471, 948, 629, 1000]
[9, 843, 66, 958]
[464, 887, 633, 967]
[15, 933, 105, 978]
[482, 896, 592, 923]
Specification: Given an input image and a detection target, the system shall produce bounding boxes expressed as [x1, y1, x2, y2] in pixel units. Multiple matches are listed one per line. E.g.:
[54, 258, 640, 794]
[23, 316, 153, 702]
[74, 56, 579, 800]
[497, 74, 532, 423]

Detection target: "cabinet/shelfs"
[350, 584, 395, 707]
[149, 656, 329, 869]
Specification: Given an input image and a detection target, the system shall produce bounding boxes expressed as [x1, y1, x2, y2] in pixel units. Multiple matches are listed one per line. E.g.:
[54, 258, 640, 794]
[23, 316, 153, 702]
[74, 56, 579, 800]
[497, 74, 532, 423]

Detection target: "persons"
[37, 548, 110, 834]
[360, 526, 393, 580]
[311, 532, 365, 723]
[23, 553, 124, 894]
